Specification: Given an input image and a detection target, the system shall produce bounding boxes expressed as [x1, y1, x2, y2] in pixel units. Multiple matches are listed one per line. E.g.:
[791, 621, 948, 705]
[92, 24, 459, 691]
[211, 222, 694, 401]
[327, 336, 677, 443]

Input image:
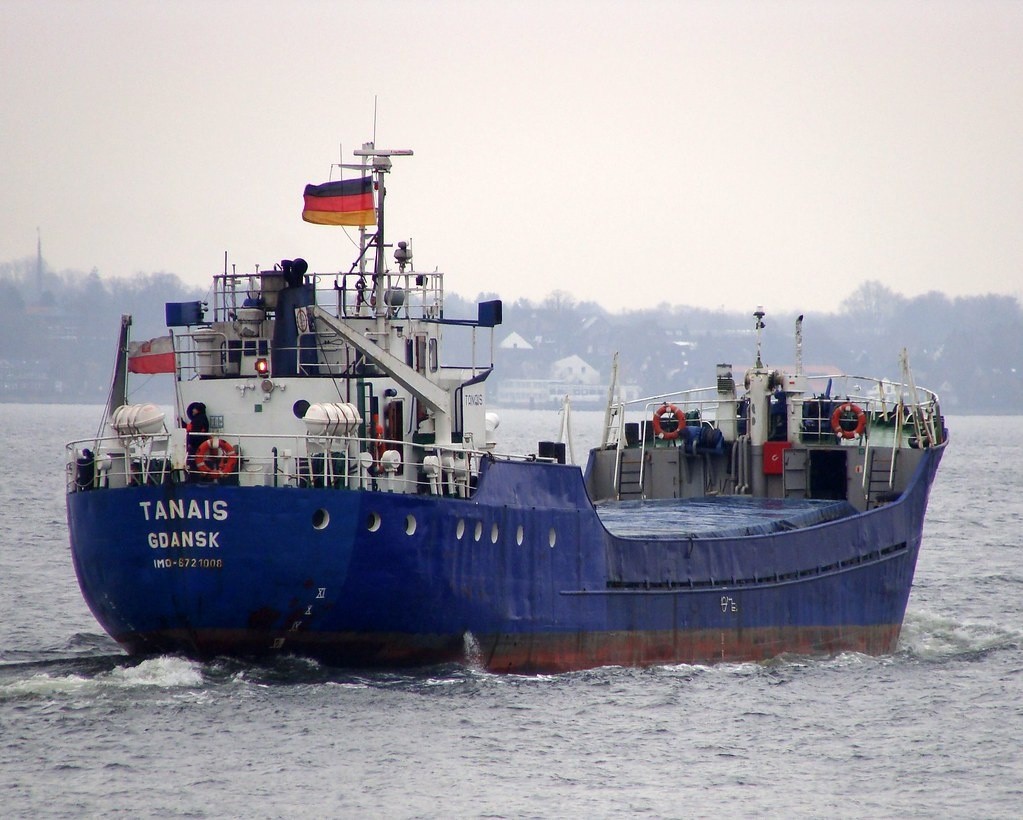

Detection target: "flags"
[302, 175, 377, 226]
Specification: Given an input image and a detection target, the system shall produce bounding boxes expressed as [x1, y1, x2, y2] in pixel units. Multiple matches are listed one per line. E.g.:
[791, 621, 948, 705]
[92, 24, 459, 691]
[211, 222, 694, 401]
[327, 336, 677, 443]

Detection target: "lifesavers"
[831, 403, 867, 439]
[195, 438, 238, 479]
[908, 428, 931, 449]
[653, 403, 686, 440]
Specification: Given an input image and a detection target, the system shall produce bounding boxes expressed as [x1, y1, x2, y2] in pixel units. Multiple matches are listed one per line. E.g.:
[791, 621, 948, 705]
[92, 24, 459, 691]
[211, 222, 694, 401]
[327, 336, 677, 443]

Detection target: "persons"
[178, 402, 209, 481]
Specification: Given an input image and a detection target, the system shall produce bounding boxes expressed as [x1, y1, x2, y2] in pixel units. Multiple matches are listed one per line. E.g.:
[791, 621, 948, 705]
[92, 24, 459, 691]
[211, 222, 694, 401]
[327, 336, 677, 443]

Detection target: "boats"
[65, 92, 952, 673]
